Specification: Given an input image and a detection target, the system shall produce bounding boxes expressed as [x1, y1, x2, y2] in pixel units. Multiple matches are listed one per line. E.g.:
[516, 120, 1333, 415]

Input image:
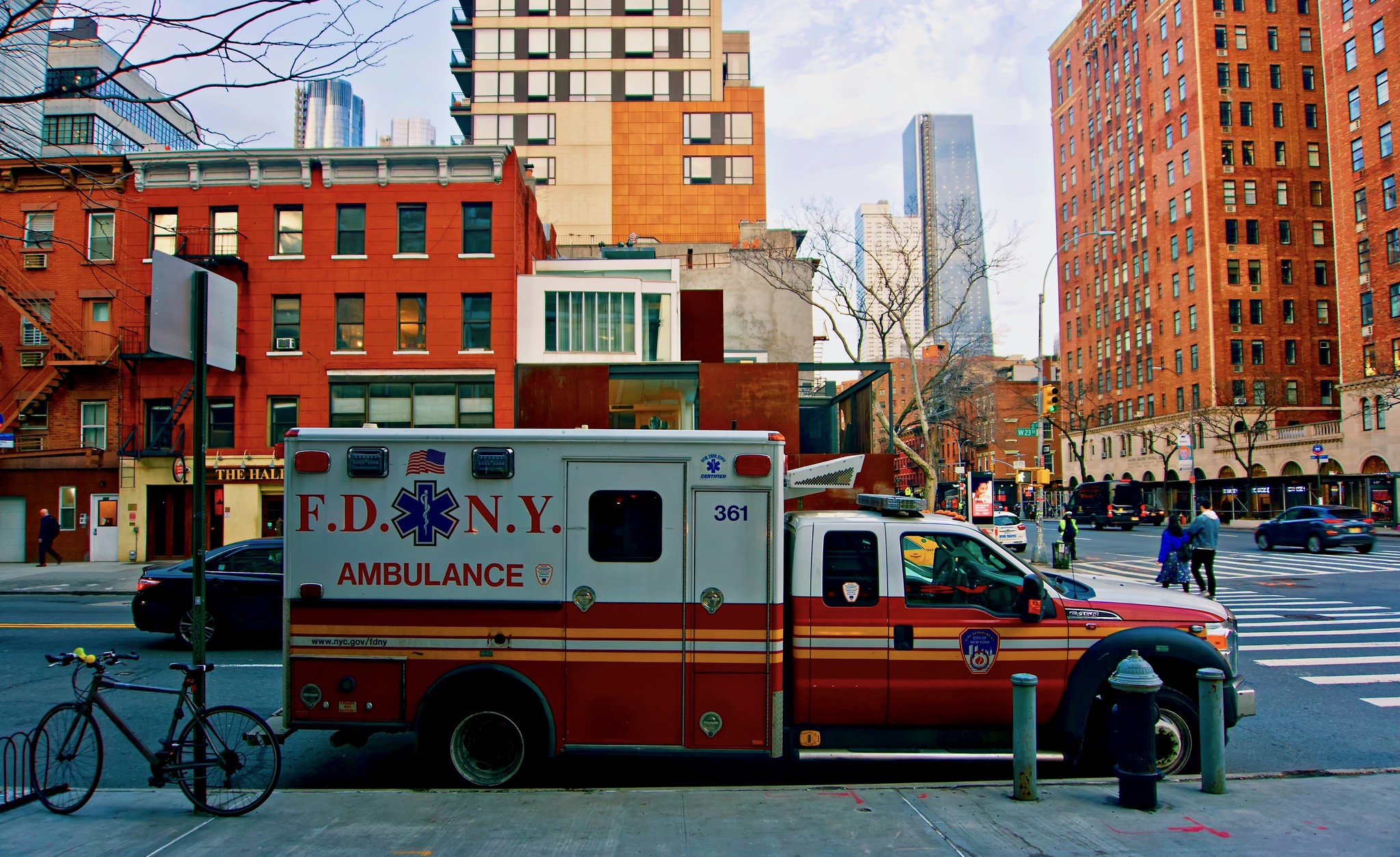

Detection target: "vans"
[1064, 479, 1145, 531]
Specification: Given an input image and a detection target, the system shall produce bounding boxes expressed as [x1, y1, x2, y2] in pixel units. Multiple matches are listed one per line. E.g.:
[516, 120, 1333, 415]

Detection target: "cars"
[1139, 504, 1165, 527]
[1253, 504, 1377, 555]
[994, 500, 1008, 512]
[981, 511, 1028, 552]
[131, 535, 284, 651]
[1012, 501, 1037, 515]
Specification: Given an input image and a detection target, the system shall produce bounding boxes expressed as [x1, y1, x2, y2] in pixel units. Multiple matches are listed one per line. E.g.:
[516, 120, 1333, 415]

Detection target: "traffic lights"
[1015, 469, 1033, 485]
[1042, 381, 1060, 414]
[1036, 468, 1050, 486]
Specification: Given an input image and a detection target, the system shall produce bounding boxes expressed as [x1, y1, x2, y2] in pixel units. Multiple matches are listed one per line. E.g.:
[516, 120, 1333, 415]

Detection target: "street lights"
[938, 427, 971, 502]
[1030, 230, 1116, 564]
[1151, 365, 1197, 527]
[991, 453, 1026, 519]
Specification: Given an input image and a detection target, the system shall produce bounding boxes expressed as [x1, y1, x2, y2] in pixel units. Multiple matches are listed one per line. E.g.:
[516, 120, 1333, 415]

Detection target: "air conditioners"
[1105, 115, 1112, 122]
[1234, 398, 1246, 404]
[1077, 411, 1084, 416]
[1064, 60, 1071, 67]
[921, 444, 925, 447]
[1133, 411, 1144, 417]
[277, 338, 296, 349]
[1084, 57, 1090, 64]
[1131, 236, 1138, 243]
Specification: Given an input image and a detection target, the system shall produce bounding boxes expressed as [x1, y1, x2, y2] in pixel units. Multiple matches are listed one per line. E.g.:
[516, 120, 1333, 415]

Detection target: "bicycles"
[28, 645, 282, 818]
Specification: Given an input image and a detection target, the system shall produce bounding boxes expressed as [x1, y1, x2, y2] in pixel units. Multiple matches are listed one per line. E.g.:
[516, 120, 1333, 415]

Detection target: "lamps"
[240, 449, 252, 468]
[213, 450, 223, 468]
[269, 450, 279, 468]
[100, 480, 106, 486]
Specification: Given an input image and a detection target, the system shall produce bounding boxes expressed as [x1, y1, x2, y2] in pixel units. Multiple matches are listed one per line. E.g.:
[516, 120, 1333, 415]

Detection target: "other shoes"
[1209, 596, 1217, 601]
[1072, 558, 1078, 560]
[1200, 591, 1211, 598]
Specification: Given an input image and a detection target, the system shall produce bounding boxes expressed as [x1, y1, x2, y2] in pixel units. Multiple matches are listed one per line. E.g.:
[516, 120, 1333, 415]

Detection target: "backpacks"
[1171, 537, 1193, 562]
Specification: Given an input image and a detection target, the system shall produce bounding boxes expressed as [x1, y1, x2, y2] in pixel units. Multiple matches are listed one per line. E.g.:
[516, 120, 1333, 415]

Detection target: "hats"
[1066, 511, 1072, 516]
[960, 501, 963, 503]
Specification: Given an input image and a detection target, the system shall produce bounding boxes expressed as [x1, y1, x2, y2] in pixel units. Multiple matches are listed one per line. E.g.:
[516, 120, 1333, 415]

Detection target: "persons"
[1156, 497, 1240, 601]
[36, 508, 62, 567]
[1026, 501, 1064, 522]
[1057, 511, 1079, 560]
[934, 500, 966, 516]
[972, 480, 991, 503]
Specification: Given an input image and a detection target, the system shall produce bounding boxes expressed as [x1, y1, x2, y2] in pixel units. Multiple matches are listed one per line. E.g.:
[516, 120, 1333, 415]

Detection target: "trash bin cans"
[1052, 542, 1072, 569]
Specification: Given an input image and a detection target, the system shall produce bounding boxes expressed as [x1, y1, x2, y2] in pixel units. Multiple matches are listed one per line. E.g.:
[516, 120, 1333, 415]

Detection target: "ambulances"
[279, 418, 1260, 776]
